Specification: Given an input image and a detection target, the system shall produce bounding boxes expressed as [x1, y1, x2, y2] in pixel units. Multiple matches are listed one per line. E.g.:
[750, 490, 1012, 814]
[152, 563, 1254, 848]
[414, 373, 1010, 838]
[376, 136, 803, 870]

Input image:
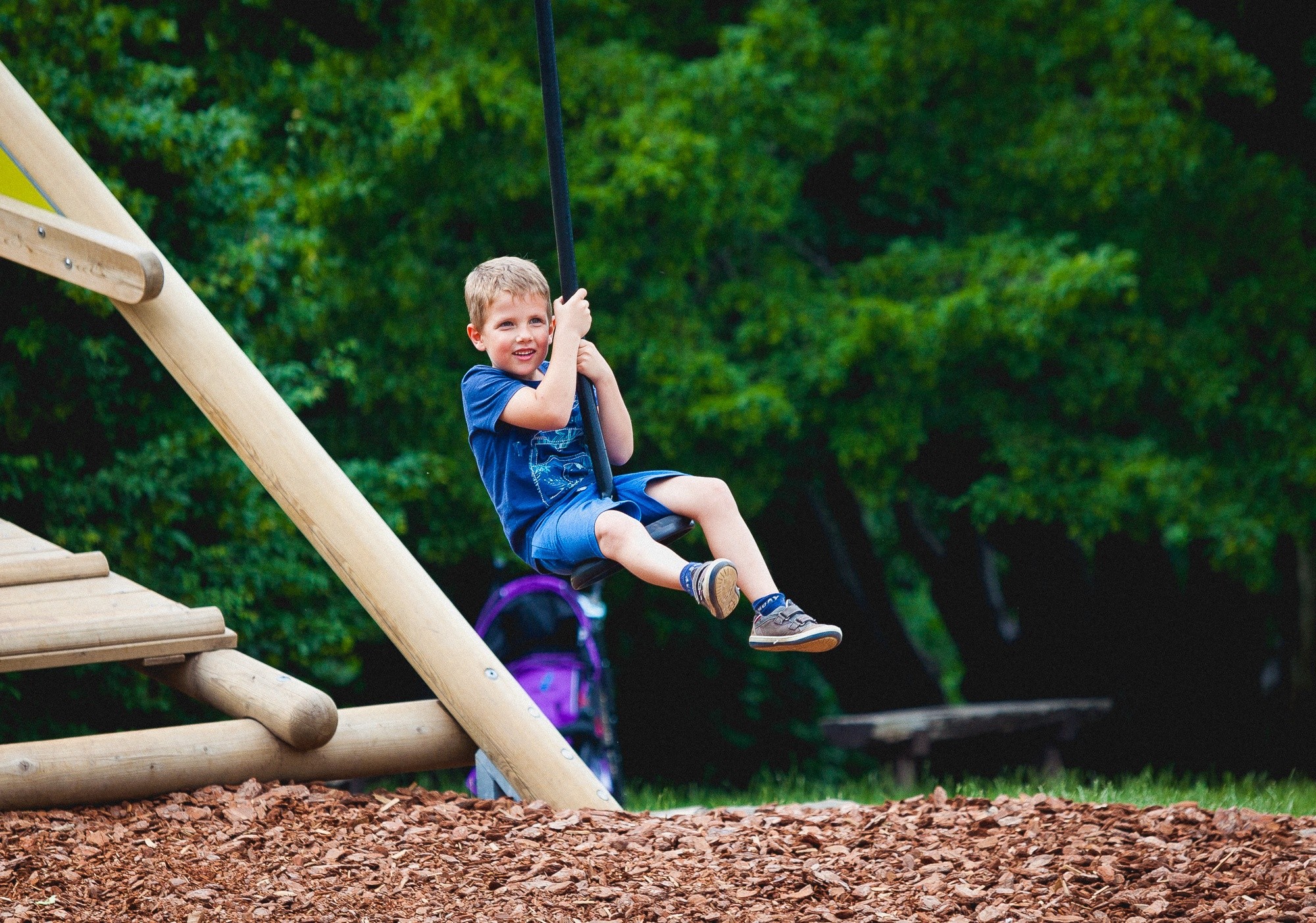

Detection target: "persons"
[461, 255, 842, 653]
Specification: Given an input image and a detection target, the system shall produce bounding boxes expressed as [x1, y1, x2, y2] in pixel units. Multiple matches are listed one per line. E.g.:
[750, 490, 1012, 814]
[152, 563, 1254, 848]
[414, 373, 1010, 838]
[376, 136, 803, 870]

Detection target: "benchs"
[813, 697, 1115, 789]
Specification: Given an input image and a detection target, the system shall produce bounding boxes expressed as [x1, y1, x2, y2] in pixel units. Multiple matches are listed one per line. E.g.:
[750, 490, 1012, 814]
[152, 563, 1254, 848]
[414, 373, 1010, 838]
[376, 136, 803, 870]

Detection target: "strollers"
[468, 577, 624, 807]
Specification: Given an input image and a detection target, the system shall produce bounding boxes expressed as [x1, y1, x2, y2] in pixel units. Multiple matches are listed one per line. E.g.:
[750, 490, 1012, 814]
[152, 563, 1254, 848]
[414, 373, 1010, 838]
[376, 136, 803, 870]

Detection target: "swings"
[535, 0, 696, 591]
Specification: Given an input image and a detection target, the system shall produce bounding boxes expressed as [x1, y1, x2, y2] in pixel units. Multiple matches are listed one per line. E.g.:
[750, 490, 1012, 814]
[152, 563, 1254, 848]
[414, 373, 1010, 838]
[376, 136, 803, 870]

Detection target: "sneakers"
[690, 558, 741, 620]
[748, 599, 843, 652]
